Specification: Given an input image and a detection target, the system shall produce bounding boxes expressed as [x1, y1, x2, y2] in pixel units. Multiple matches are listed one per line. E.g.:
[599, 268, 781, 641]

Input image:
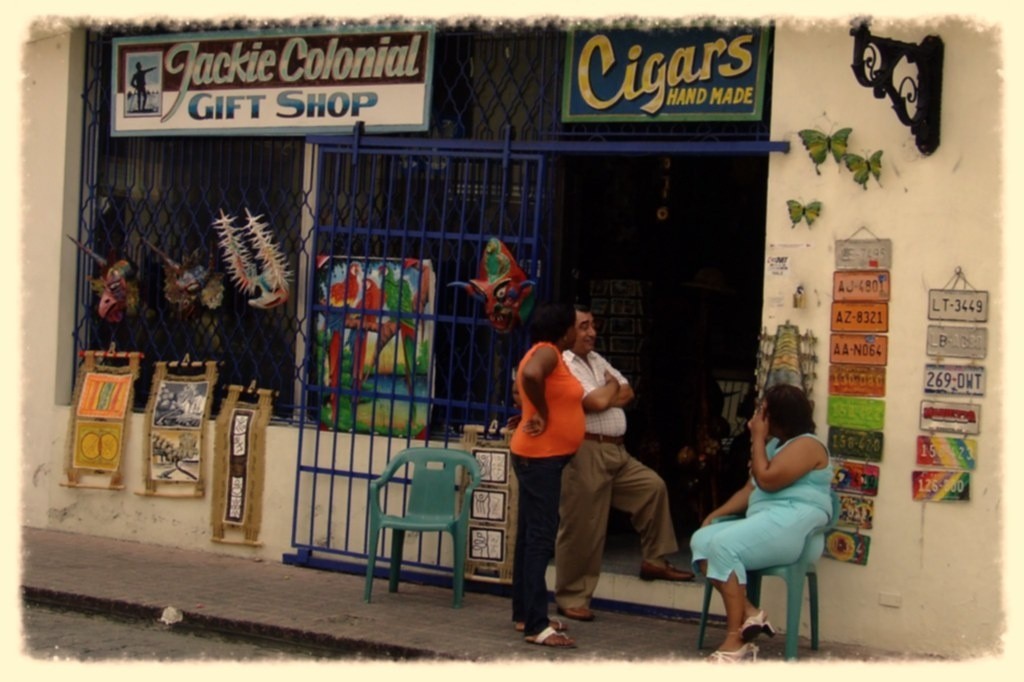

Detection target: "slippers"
[513, 620, 568, 632]
[525, 625, 578, 648]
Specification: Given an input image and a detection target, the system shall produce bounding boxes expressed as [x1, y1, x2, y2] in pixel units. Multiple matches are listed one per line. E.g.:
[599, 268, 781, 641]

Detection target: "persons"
[688, 382, 834, 664]
[553, 302, 698, 620]
[507, 300, 588, 648]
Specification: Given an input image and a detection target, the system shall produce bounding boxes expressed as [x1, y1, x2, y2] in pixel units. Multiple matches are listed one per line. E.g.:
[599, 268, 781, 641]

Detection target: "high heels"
[739, 608, 776, 640]
[704, 638, 759, 662]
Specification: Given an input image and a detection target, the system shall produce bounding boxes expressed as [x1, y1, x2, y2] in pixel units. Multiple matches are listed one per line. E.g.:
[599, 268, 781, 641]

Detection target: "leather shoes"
[638, 560, 696, 581]
[582, 432, 624, 447]
[558, 605, 597, 621]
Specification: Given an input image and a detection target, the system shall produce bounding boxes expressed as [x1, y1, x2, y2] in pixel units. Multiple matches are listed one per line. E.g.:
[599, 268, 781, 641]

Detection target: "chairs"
[365, 444, 481, 609]
[696, 490, 840, 660]
